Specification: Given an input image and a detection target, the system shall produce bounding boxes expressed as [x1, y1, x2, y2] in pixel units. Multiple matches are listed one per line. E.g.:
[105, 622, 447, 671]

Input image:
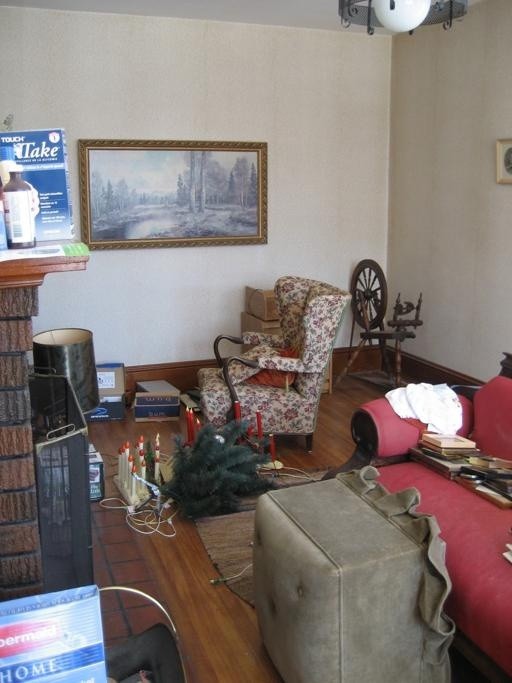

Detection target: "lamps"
[33, 328, 100, 437]
[336, 0, 468, 36]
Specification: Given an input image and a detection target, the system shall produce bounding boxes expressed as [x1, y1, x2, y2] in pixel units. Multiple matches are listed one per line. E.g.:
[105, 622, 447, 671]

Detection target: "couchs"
[351, 352, 511, 683]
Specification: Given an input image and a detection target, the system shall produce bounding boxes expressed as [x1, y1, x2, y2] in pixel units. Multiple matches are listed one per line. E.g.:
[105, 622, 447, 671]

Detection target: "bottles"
[3, 171, 40, 248]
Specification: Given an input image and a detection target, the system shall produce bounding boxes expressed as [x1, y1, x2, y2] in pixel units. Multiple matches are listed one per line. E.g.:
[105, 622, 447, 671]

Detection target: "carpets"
[194, 468, 340, 608]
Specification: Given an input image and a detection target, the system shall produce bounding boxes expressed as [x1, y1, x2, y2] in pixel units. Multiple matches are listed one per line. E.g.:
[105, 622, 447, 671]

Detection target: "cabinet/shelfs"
[241, 285, 333, 395]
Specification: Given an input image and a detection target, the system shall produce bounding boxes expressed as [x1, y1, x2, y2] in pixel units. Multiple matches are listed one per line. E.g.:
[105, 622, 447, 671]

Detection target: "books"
[500, 526, 512, 563]
[406, 431, 512, 508]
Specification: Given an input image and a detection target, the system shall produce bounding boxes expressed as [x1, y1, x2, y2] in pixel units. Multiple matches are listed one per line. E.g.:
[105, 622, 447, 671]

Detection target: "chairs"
[196, 275, 353, 454]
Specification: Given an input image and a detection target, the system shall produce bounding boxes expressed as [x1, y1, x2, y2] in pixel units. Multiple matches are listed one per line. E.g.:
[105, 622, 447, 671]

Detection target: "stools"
[253, 464, 456, 682]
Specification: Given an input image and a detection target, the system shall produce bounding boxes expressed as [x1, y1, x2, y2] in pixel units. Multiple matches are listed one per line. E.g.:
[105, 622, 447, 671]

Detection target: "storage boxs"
[135, 380, 181, 392]
[89, 362, 126, 396]
[129, 377, 185, 422]
[81, 396, 126, 422]
[89, 443, 105, 503]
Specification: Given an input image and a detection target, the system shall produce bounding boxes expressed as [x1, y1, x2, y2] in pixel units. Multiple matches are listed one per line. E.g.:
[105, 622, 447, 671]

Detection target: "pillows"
[244, 349, 299, 387]
[215, 340, 280, 385]
[470, 374, 511, 460]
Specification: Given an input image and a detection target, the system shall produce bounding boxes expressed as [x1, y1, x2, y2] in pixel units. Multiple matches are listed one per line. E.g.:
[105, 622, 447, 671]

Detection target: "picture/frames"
[494, 137, 512, 186]
[78, 138, 268, 251]
